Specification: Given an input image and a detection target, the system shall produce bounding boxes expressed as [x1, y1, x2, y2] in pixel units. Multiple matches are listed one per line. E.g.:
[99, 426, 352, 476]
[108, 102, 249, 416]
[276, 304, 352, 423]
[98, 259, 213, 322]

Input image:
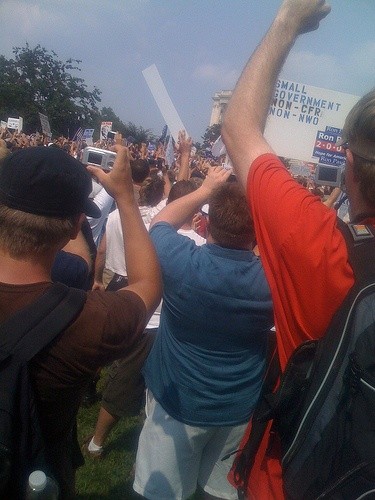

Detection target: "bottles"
[24, 469, 58, 500]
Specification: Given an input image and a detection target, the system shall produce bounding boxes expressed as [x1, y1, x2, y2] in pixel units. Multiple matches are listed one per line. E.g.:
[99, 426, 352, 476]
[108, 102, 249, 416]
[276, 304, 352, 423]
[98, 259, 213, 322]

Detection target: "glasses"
[342, 141, 350, 150]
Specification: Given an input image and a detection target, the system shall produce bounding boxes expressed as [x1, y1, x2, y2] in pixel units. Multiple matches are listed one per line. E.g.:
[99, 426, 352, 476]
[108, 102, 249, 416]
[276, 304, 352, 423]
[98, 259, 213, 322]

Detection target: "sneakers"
[77, 427, 104, 463]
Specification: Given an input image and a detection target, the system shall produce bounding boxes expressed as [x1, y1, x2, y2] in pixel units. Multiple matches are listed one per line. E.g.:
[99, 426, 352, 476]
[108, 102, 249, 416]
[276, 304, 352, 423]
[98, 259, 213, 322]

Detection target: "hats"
[0, 145, 103, 219]
[334, 137, 375, 163]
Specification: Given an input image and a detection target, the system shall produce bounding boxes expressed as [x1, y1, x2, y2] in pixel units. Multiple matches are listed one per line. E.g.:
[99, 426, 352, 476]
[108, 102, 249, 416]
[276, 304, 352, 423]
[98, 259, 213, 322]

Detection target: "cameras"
[314, 162, 348, 193]
[106, 131, 116, 146]
[223, 165, 236, 176]
[80, 146, 117, 171]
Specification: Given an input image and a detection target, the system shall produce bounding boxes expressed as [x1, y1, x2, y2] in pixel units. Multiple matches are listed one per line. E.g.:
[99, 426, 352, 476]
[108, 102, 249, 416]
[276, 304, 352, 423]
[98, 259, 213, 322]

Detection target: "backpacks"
[221, 216, 375, 500]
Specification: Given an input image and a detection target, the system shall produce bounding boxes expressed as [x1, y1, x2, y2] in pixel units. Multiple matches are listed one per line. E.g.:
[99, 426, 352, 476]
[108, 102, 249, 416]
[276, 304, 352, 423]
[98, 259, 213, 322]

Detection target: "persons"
[133, 166, 273, 500]
[220, 0, 375, 500]
[0, 144, 163, 500]
[0, 126, 353, 460]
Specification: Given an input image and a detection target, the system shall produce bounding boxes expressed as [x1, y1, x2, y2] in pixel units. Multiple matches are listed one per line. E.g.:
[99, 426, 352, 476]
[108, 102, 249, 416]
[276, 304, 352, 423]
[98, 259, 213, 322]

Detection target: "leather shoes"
[80, 376, 103, 409]
